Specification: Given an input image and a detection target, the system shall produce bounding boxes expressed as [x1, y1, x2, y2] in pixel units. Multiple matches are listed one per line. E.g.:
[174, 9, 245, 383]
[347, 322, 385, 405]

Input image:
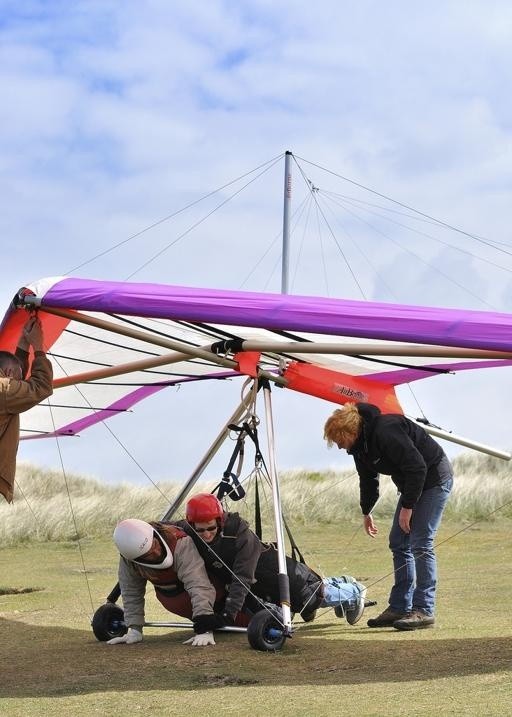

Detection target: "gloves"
[107, 628, 142, 645]
[182, 630, 216, 647]
[193, 610, 234, 635]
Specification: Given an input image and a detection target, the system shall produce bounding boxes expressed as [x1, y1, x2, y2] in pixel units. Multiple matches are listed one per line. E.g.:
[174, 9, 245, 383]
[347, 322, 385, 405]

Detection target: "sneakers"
[347, 583, 367, 624]
[394, 610, 437, 630]
[368, 606, 409, 628]
[335, 575, 356, 618]
[302, 610, 315, 622]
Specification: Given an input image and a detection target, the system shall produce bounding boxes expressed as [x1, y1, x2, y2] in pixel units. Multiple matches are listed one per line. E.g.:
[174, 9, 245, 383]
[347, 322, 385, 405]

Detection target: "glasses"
[192, 523, 217, 533]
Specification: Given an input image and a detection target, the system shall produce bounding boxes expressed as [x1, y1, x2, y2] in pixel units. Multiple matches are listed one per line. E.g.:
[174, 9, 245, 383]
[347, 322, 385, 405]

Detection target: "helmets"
[113, 519, 174, 570]
[186, 493, 224, 529]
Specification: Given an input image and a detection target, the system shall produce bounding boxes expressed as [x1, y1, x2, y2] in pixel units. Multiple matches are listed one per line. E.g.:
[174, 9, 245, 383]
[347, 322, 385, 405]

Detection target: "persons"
[322, 399, 456, 631]
[153, 490, 368, 634]
[0, 314, 57, 505]
[104, 517, 258, 647]
